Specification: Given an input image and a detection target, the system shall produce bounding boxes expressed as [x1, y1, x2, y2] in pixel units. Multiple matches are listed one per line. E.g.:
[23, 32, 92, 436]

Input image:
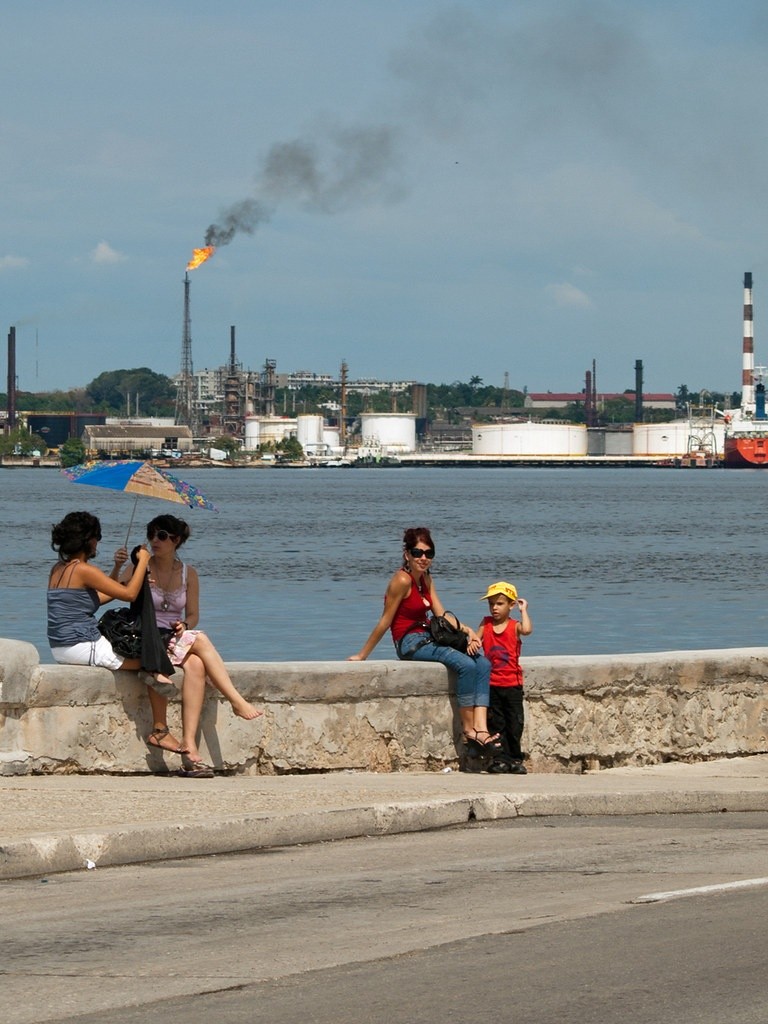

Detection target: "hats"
[481, 582, 517, 602]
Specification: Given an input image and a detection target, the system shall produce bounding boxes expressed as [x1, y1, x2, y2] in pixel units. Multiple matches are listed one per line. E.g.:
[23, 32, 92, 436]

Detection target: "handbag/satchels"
[430, 611, 469, 654]
[96, 607, 181, 659]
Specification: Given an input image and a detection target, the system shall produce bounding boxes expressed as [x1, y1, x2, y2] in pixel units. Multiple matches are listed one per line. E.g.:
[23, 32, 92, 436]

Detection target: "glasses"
[410, 548, 435, 559]
[96, 534, 102, 541]
[147, 528, 175, 541]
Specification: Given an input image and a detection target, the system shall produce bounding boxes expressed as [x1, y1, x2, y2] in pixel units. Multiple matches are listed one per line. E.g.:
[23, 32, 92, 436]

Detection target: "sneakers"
[487, 761, 526, 774]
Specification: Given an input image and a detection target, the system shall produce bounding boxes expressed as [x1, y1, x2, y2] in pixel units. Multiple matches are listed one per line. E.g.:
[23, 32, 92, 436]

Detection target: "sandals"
[461, 728, 501, 752]
[137, 671, 177, 697]
[146, 726, 190, 754]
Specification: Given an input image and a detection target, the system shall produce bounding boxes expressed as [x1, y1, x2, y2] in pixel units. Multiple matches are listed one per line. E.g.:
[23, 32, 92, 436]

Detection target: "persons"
[466, 581, 532, 774]
[347, 527, 504, 759]
[46, 510, 191, 756]
[102, 513, 263, 760]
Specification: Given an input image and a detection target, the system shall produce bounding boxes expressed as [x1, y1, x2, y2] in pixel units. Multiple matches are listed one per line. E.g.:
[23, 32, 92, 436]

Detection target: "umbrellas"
[58, 458, 219, 550]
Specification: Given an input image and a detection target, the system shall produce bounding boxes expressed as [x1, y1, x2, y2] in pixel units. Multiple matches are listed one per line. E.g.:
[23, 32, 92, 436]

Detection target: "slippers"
[179, 762, 214, 778]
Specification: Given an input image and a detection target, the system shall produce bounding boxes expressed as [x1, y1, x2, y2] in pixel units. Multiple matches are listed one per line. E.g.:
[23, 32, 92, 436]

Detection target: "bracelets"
[183, 621, 189, 629]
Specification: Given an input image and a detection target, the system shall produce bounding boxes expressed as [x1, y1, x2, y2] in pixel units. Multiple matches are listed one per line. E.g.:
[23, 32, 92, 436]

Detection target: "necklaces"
[152, 558, 177, 611]
[419, 585, 422, 591]
